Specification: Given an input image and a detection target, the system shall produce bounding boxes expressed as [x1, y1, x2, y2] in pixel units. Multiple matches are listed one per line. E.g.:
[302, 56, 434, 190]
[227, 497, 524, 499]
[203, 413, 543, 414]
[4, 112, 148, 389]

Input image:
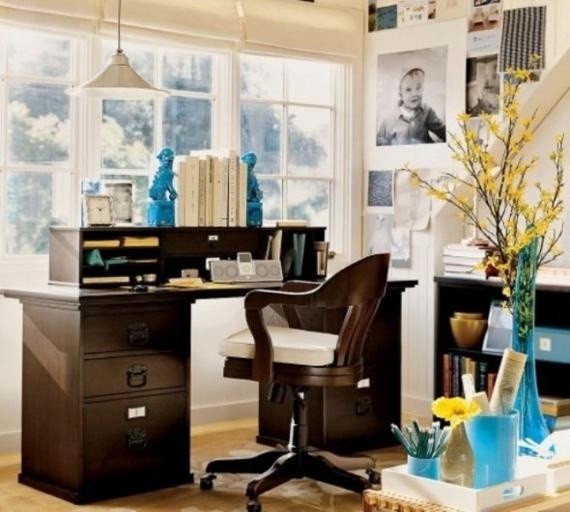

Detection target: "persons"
[242, 152, 264, 203]
[377, 66, 446, 143]
[149, 145, 177, 202]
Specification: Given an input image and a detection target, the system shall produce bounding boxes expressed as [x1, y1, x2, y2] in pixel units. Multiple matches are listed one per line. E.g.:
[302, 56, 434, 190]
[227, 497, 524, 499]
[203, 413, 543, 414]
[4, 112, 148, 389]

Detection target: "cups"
[453, 311, 482, 319]
[407, 453, 441, 480]
[469, 408, 520, 489]
[450, 319, 486, 349]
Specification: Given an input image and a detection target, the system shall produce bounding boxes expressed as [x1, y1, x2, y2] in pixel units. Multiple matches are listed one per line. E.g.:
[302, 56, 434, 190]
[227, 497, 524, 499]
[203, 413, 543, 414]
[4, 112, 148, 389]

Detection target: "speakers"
[209, 260, 283, 283]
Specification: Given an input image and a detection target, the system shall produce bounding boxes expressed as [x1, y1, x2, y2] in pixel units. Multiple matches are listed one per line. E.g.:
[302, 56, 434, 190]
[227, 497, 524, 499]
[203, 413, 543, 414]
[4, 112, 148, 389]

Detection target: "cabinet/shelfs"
[430, 272, 568, 429]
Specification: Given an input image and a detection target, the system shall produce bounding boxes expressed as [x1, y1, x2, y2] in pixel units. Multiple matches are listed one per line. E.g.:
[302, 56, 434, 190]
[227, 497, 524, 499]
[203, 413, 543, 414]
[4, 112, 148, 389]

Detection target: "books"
[174, 145, 248, 228]
[443, 244, 507, 283]
[314, 241, 329, 276]
[83, 274, 131, 285]
[314, 249, 321, 275]
[83, 238, 121, 249]
[292, 233, 306, 278]
[121, 236, 160, 247]
[443, 352, 570, 431]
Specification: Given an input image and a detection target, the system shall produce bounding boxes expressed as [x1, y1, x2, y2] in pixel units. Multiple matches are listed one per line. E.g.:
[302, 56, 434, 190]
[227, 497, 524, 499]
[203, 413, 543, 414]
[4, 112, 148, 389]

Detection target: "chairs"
[202, 253, 391, 512]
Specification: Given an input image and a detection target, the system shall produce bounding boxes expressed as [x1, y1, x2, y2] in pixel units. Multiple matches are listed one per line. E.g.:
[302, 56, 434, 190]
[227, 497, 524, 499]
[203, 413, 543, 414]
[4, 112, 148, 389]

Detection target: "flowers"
[396, 53, 569, 313]
[431, 388, 481, 428]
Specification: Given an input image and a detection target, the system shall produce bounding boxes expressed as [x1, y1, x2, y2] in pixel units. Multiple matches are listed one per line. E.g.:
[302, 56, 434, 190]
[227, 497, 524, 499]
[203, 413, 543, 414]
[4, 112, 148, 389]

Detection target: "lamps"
[67, 0, 170, 100]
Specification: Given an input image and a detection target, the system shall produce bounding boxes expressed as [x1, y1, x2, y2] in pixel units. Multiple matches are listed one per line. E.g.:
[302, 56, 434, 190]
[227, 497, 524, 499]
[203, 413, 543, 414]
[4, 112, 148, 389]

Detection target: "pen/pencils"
[391, 420, 450, 458]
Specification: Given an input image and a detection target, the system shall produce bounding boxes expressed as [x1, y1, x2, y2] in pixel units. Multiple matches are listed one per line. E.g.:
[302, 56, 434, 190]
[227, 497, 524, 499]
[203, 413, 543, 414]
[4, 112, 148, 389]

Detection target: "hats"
[398, 60, 425, 98]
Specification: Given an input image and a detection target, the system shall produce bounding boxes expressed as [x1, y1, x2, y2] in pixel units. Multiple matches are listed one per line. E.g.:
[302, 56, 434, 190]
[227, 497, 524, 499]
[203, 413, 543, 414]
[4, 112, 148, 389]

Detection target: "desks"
[0, 226, 418, 506]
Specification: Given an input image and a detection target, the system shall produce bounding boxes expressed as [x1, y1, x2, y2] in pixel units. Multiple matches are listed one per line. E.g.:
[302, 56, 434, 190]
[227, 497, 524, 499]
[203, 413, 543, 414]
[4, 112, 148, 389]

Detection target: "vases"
[504, 231, 554, 455]
[438, 423, 475, 485]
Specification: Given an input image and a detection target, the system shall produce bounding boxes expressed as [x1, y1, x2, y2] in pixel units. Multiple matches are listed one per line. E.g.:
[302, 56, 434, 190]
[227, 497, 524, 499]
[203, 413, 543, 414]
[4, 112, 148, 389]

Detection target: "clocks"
[83, 195, 115, 225]
